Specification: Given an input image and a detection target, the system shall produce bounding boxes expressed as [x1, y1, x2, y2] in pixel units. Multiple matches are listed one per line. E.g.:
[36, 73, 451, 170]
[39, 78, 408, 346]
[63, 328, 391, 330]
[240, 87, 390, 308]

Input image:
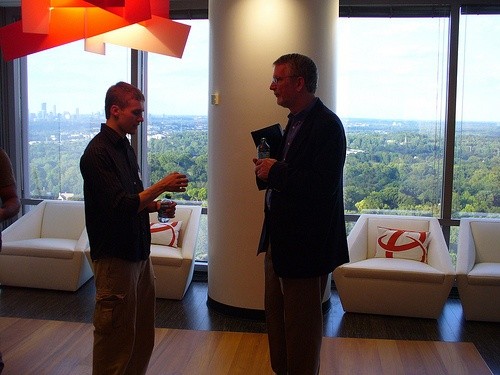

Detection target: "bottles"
[158, 194, 174, 225]
[256, 136, 273, 161]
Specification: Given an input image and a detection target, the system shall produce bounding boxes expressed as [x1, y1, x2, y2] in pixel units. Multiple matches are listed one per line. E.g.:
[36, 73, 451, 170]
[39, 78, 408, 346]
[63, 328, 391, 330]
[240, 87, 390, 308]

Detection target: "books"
[251, 123, 282, 160]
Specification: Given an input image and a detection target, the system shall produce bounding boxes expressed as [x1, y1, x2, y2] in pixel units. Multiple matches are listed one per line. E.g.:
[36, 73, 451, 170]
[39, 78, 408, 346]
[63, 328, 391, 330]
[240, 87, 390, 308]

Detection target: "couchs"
[0, 200, 94, 292]
[456, 218, 500, 321]
[334, 214, 456, 317]
[85, 205, 202, 299]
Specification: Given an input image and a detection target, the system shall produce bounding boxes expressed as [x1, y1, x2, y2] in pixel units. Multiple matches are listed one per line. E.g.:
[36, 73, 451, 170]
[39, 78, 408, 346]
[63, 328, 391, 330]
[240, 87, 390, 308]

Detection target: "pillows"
[374, 225, 430, 263]
[149, 220, 183, 248]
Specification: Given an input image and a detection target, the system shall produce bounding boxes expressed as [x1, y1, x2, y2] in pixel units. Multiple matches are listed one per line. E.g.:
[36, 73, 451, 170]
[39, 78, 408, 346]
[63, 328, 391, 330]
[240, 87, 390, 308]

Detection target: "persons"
[252, 53, 350, 375]
[79, 82, 189, 375]
[0, 147, 21, 253]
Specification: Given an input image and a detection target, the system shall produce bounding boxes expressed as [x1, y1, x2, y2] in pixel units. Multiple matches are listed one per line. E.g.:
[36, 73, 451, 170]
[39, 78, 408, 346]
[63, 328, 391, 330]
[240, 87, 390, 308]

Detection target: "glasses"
[273, 77, 296, 83]
